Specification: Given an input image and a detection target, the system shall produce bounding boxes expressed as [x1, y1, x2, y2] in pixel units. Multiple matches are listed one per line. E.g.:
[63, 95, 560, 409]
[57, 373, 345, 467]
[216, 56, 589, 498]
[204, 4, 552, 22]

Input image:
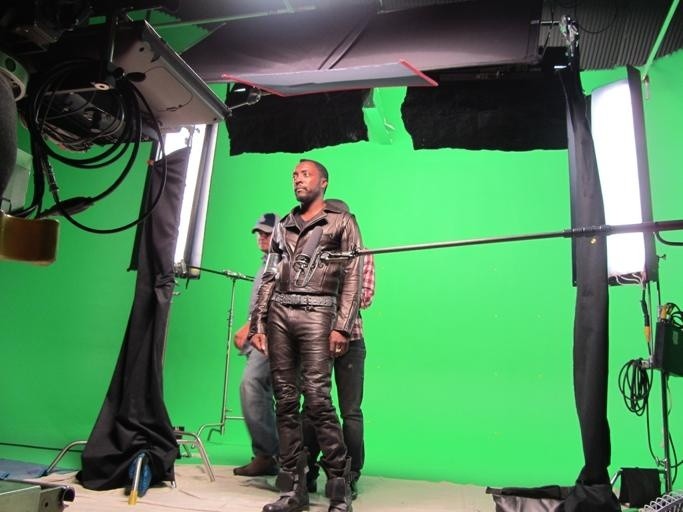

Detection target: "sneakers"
[233, 457, 278, 476]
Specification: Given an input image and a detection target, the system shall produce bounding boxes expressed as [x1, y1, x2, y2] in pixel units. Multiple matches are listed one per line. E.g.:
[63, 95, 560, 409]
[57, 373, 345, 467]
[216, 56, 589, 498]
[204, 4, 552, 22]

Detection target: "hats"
[251, 212, 280, 234]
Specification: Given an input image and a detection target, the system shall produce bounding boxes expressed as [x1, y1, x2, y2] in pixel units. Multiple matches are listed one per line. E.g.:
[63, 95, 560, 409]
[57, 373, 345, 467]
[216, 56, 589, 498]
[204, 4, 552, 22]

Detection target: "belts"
[270, 292, 336, 307]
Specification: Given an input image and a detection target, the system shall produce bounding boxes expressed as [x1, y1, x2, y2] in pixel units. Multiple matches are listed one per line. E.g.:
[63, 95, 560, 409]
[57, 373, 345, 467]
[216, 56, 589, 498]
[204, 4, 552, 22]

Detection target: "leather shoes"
[307, 480, 316, 493]
[349, 481, 356, 500]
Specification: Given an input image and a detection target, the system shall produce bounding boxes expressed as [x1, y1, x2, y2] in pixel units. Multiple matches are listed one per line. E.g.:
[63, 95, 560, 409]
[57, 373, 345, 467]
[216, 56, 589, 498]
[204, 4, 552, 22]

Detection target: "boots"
[262, 448, 309, 512]
[314, 455, 352, 512]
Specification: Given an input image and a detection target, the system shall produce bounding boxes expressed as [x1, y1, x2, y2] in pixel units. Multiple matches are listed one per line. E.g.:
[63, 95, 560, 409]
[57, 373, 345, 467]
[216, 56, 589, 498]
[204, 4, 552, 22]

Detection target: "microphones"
[181, 260, 187, 274]
[294, 226, 323, 281]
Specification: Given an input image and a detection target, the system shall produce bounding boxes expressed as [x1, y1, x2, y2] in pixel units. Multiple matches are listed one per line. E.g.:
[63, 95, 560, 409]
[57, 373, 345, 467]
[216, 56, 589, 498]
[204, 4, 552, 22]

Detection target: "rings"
[335, 348, 342, 353]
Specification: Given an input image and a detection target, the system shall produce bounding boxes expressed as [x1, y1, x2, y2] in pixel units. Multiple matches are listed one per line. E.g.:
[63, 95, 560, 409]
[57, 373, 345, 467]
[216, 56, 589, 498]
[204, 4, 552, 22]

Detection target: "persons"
[233, 211, 280, 477]
[245, 158, 364, 512]
[299, 198, 376, 503]
